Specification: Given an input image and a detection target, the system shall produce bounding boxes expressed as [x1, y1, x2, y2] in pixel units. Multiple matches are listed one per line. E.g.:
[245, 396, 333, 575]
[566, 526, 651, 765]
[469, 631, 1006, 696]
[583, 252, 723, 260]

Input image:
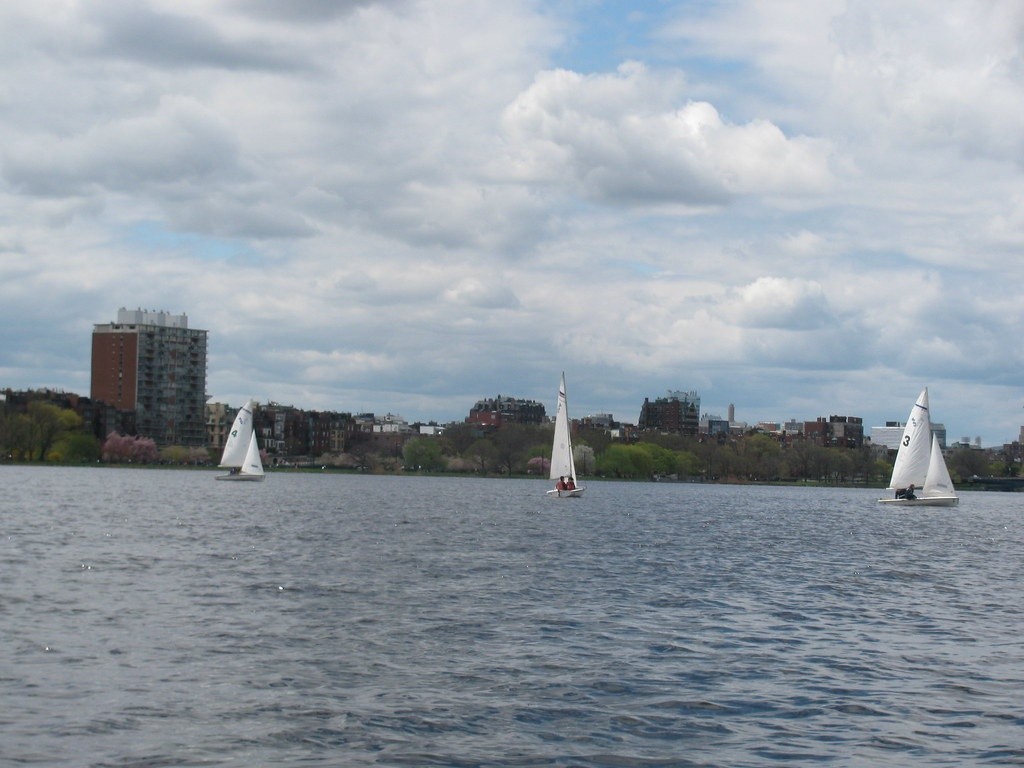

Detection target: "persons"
[895, 489, 906, 499]
[566, 477, 575, 490]
[907, 484, 918, 500]
[556, 476, 566, 490]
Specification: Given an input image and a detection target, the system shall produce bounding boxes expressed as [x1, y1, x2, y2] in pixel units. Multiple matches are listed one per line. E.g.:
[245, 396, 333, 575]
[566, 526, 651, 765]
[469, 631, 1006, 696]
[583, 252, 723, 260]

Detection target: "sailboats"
[877, 385, 960, 507]
[545, 371, 587, 499]
[216, 397, 267, 483]
